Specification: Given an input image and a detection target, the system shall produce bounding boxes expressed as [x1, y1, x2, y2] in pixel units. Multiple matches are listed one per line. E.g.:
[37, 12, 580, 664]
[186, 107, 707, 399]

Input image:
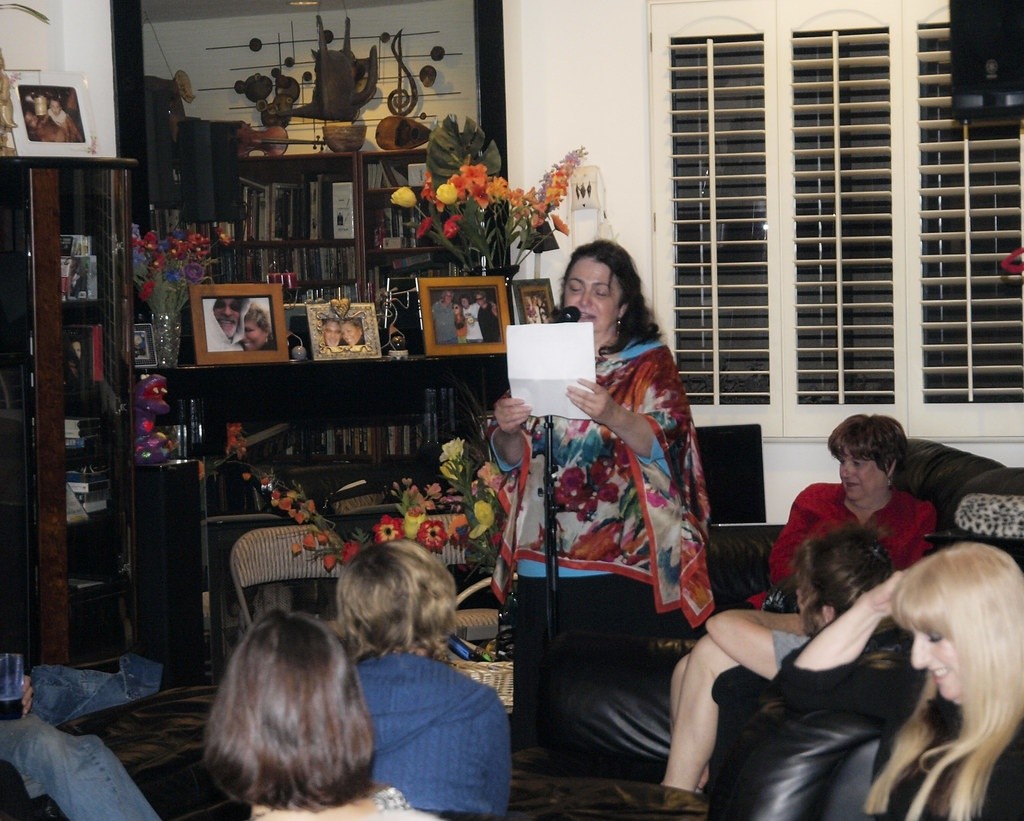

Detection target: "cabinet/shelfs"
[0, 156, 139, 677]
[145, 79, 492, 684]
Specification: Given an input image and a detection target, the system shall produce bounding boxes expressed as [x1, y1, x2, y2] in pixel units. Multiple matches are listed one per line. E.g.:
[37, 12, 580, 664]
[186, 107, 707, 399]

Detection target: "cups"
[0, 652, 25, 719]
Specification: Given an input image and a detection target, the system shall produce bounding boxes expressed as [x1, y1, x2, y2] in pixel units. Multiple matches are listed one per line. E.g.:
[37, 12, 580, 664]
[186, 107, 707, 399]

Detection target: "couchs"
[640, 440, 1004, 684]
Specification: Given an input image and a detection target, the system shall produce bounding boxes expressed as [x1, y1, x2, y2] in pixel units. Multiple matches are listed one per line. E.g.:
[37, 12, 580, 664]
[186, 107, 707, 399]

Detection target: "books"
[372, 205, 422, 248]
[150, 174, 265, 242]
[266, 173, 355, 241]
[289, 247, 356, 281]
[440, 261, 465, 277]
[213, 246, 286, 283]
[260, 425, 421, 456]
[368, 160, 408, 189]
[292, 283, 358, 302]
[367, 252, 439, 303]
[61, 235, 97, 301]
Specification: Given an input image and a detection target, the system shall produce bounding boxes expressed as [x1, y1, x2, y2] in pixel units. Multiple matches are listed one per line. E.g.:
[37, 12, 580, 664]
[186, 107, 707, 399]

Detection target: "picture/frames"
[187, 283, 290, 366]
[512, 279, 558, 325]
[3, 71, 102, 157]
[418, 277, 511, 356]
[306, 299, 383, 360]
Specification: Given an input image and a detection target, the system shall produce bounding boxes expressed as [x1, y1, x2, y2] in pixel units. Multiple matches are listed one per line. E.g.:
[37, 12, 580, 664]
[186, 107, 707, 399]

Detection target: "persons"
[0, 653, 162, 820]
[322, 318, 365, 346]
[213, 297, 274, 351]
[431, 291, 500, 344]
[662, 413, 1024, 821]
[203, 539, 509, 820]
[24, 97, 84, 142]
[485, 237, 716, 753]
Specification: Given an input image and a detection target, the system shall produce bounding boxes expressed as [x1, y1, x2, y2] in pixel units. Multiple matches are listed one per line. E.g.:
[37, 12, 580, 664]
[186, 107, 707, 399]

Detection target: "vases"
[149, 311, 180, 367]
[466, 265, 522, 325]
[478, 563, 518, 647]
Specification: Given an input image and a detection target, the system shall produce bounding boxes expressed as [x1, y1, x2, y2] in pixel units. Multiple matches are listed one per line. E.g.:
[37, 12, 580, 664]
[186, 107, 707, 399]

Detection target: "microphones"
[552, 306, 581, 323]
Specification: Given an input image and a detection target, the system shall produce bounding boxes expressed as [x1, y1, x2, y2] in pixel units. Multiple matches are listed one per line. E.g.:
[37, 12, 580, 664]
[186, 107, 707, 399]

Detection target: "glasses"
[475, 296, 485, 301]
[452, 307, 459, 309]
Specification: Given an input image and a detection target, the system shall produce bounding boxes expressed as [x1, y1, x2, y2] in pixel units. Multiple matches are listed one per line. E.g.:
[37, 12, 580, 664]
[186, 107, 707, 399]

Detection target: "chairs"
[399, 512, 501, 641]
[229, 525, 351, 639]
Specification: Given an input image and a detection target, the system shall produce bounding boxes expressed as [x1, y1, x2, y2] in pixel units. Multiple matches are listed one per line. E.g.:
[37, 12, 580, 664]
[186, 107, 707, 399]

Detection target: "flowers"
[435, 368, 510, 565]
[132, 222, 230, 324]
[389, 112, 587, 271]
[217, 422, 450, 568]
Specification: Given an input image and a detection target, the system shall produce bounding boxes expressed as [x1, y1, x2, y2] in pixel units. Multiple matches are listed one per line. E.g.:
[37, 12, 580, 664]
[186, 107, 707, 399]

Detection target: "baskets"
[438, 648, 515, 708]
